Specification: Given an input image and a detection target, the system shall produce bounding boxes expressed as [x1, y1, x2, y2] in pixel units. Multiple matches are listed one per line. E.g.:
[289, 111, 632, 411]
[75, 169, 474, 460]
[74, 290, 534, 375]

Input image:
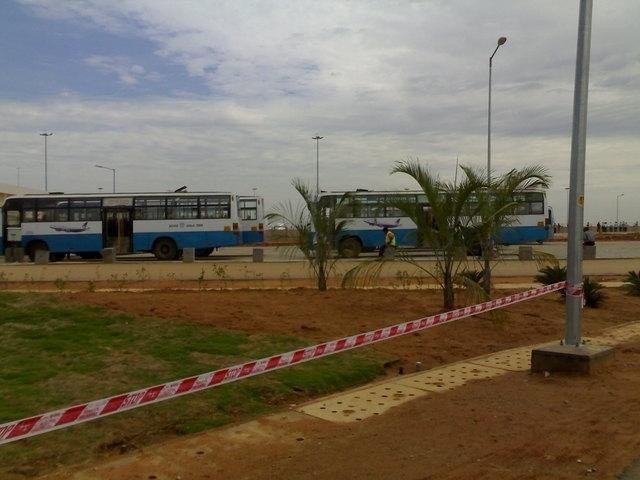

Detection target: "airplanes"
[48, 223, 91, 233]
[363, 217, 402, 229]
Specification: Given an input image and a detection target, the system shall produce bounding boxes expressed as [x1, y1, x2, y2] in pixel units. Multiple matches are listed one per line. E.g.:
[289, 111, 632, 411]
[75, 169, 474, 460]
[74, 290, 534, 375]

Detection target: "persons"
[378, 228, 396, 257]
[553, 222, 562, 233]
[583, 221, 627, 246]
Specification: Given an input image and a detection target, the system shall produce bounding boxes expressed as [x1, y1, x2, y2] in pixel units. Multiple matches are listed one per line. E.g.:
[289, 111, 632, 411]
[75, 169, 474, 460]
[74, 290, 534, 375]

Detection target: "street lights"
[565, 187, 570, 205]
[617, 193, 624, 222]
[486, 32, 507, 199]
[40, 132, 53, 192]
[95, 165, 117, 193]
[312, 135, 325, 196]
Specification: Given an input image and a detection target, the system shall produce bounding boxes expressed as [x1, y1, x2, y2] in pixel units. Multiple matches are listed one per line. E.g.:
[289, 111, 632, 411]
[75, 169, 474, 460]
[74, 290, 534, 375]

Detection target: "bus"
[307, 187, 549, 259]
[0, 189, 265, 262]
